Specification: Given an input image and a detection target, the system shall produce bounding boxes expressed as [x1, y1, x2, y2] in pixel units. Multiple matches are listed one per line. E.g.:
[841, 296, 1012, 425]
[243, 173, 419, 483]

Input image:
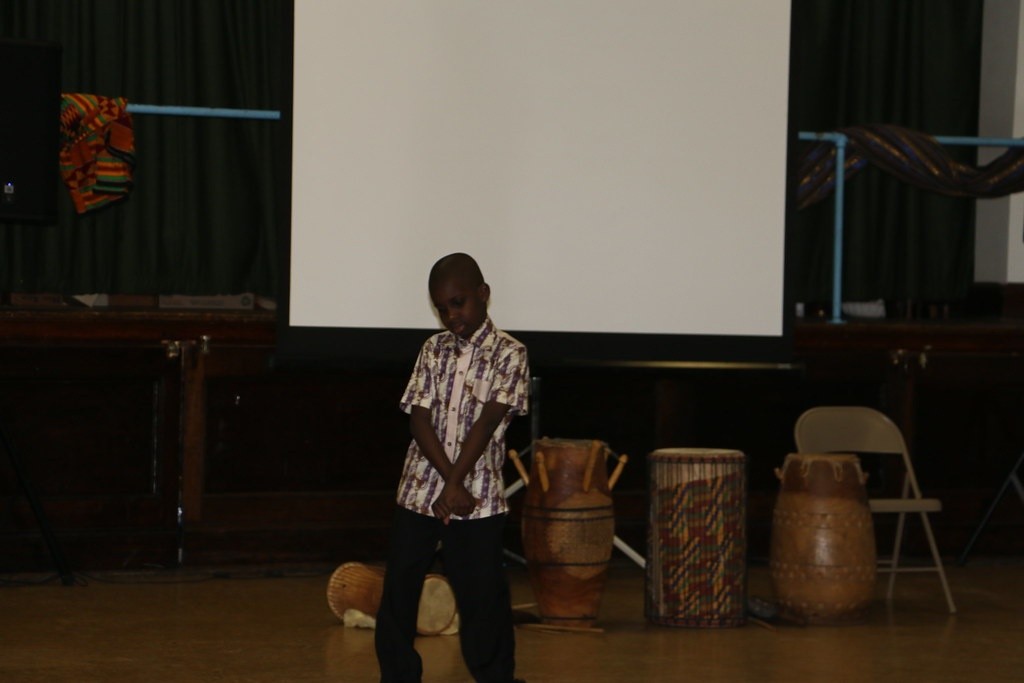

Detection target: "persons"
[375, 253, 530, 683]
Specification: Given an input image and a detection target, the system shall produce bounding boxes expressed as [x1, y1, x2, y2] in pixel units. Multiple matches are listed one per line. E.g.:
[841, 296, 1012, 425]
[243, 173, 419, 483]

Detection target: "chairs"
[794, 407, 958, 616]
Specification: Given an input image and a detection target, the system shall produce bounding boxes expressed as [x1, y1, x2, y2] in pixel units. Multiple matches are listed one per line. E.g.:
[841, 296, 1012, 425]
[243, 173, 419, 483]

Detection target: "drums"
[767, 451, 879, 625]
[325, 561, 457, 636]
[644, 447, 750, 629]
[508, 436, 628, 627]
